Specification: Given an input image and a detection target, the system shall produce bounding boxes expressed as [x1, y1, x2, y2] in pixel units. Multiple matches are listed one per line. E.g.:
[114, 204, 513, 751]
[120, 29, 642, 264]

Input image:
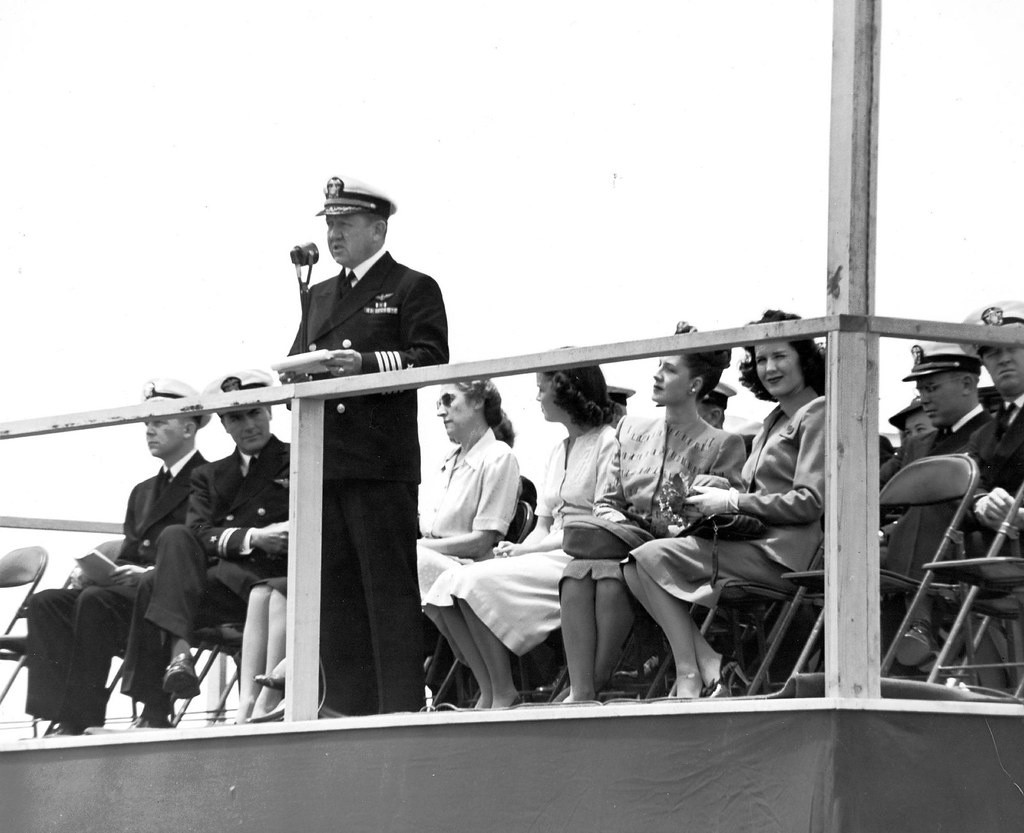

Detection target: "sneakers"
[611, 643, 658, 679]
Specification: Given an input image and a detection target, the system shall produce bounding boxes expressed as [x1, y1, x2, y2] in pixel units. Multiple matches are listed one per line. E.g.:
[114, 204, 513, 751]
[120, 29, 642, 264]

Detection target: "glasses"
[436, 393, 456, 409]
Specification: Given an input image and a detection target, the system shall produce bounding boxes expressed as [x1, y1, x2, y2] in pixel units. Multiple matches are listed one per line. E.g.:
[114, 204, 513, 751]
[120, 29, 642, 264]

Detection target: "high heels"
[701, 654, 752, 699]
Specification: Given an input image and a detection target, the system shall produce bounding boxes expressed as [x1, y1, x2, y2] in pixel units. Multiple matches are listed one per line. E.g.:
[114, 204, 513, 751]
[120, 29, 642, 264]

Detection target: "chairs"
[0, 542, 247, 737]
[424, 453, 1024, 702]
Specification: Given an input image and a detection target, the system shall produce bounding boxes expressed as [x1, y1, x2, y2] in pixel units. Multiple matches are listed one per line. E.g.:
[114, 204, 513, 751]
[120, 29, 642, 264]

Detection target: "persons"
[416, 346, 635, 711]
[121, 370, 290, 728]
[879, 301, 1024, 704]
[25, 378, 212, 737]
[278, 176, 450, 720]
[551, 311, 825, 702]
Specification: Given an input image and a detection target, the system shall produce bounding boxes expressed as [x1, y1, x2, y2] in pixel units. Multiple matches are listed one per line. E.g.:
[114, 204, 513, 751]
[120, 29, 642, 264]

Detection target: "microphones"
[290, 243, 320, 265]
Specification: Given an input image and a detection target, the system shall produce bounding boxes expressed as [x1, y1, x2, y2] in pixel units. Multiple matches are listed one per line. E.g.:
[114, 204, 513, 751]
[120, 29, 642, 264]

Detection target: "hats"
[315, 174, 398, 221]
[955, 299, 1024, 359]
[889, 395, 923, 431]
[903, 339, 982, 382]
[606, 385, 637, 406]
[656, 381, 737, 410]
[201, 368, 272, 416]
[140, 380, 211, 430]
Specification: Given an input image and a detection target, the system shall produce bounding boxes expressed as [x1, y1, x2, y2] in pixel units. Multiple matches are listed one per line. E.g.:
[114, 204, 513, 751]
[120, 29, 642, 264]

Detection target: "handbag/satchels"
[675, 511, 767, 541]
[563, 511, 655, 560]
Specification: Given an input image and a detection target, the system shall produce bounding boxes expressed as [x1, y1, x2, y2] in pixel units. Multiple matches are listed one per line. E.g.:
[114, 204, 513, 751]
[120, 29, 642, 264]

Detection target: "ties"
[247, 455, 258, 475]
[338, 271, 355, 302]
[995, 402, 1019, 444]
[154, 467, 172, 504]
[929, 425, 953, 455]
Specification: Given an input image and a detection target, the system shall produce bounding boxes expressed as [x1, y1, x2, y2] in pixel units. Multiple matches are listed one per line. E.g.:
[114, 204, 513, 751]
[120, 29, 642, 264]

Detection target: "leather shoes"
[532, 663, 569, 695]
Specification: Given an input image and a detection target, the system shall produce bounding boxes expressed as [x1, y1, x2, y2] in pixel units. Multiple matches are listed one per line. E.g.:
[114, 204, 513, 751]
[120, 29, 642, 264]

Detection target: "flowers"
[661, 471, 688, 528]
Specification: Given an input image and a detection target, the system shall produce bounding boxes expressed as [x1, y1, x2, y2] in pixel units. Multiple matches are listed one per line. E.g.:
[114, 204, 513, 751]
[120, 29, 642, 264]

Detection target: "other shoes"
[894, 624, 942, 672]
[253, 672, 285, 691]
[511, 693, 523, 706]
[43, 718, 88, 737]
[163, 651, 200, 698]
[129, 715, 174, 728]
